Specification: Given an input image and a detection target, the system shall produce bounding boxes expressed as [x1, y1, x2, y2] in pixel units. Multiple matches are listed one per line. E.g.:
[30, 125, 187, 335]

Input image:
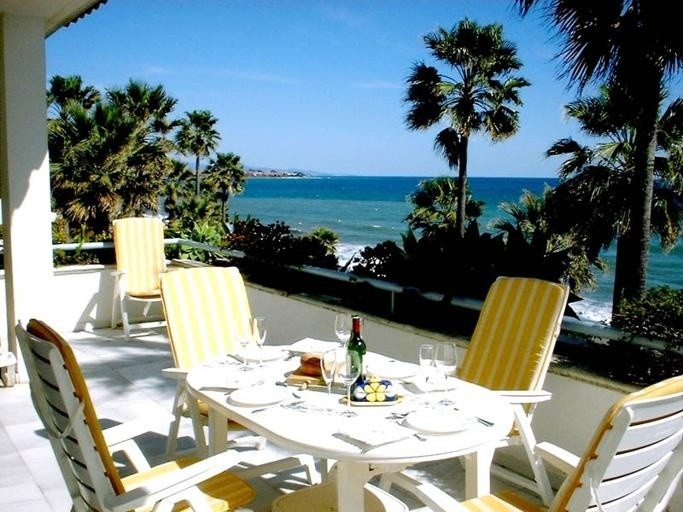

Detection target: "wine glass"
[419, 342, 457, 405]
[320, 349, 361, 418]
[236, 317, 268, 371]
[334, 314, 351, 345]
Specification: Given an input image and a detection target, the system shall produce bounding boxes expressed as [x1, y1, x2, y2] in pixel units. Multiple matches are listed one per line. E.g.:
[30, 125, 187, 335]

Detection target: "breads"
[300, 355, 322, 377]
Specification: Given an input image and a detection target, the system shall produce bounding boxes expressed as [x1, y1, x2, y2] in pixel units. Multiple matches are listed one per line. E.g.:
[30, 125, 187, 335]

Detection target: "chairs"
[463, 375, 683, 512]
[453, 275, 570, 507]
[159, 266, 265, 457]
[13, 318, 255, 511]
[110, 217, 166, 340]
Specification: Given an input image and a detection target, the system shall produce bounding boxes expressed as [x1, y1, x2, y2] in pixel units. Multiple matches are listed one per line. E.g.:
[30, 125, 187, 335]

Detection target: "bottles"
[347, 316, 367, 381]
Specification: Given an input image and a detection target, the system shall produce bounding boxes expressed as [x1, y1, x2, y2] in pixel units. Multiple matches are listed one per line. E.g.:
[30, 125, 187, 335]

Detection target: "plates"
[230, 385, 287, 405]
[372, 361, 417, 379]
[334, 422, 419, 447]
[236, 344, 281, 361]
[406, 408, 473, 433]
[340, 392, 405, 406]
